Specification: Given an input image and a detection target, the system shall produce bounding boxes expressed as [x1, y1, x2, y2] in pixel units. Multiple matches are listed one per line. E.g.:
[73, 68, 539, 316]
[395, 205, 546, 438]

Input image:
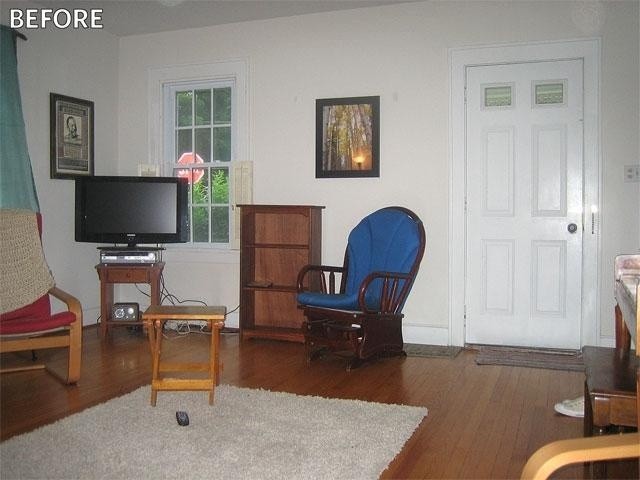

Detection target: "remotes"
[176, 411, 189, 424]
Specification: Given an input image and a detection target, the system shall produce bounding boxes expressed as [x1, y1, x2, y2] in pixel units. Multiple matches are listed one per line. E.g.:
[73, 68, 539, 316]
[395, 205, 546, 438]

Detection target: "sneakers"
[555, 396, 585, 418]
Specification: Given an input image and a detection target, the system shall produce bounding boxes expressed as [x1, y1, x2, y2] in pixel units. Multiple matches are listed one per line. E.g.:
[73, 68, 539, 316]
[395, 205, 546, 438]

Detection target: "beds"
[613, 254, 640, 388]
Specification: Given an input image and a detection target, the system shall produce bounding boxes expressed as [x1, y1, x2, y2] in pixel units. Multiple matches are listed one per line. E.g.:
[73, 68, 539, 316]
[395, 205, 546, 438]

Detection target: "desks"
[95, 261, 164, 342]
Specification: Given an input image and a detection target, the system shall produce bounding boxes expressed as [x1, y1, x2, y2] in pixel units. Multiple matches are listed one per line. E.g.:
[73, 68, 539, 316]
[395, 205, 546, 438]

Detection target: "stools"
[582, 339, 640, 480]
[142, 305, 226, 407]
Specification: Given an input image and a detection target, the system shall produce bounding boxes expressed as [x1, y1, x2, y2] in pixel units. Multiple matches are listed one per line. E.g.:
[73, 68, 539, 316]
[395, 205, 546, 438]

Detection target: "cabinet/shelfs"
[235, 203, 325, 349]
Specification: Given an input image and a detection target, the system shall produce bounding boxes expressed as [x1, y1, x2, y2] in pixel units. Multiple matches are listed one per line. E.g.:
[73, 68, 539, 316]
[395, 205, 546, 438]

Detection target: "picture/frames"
[315, 95, 380, 179]
[49, 93, 95, 180]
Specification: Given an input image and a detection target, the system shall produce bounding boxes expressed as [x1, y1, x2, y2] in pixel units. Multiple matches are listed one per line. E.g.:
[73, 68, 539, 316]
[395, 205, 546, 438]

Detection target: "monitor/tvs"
[74, 174, 190, 251]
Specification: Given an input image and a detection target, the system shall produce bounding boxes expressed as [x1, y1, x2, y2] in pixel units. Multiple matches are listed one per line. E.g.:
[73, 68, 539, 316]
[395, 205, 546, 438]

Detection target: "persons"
[64, 114, 84, 143]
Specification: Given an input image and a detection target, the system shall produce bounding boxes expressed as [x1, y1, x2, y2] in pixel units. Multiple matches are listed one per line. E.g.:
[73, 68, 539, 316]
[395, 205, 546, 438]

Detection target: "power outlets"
[623, 165, 640, 181]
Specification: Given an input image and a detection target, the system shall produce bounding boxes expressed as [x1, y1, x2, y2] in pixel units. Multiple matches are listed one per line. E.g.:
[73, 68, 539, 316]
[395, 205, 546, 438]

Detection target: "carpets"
[0, 384, 427, 479]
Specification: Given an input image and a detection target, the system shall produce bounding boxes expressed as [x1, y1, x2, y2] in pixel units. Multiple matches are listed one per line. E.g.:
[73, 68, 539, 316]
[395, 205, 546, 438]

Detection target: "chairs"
[0, 206, 82, 384]
[296, 205, 426, 370]
